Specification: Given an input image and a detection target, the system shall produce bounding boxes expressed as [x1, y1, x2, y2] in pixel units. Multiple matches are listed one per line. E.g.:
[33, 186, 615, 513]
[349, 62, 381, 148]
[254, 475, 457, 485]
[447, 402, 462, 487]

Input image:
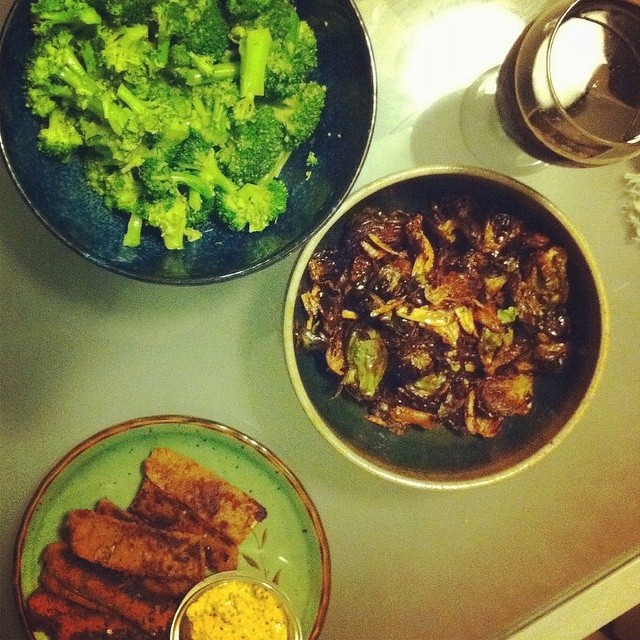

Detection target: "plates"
[11, 414, 332, 640]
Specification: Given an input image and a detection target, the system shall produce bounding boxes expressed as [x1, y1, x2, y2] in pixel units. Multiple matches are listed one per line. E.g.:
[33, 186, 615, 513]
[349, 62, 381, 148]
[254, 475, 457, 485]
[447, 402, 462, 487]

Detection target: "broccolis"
[17, 0, 327, 251]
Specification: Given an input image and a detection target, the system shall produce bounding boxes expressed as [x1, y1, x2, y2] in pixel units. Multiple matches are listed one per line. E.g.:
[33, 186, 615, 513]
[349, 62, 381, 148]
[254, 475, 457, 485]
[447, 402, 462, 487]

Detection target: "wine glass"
[460, 0, 639, 175]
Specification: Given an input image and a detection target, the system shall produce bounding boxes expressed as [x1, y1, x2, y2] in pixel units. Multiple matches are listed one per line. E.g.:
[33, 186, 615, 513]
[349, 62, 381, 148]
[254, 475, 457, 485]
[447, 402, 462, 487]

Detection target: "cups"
[169, 571, 302, 640]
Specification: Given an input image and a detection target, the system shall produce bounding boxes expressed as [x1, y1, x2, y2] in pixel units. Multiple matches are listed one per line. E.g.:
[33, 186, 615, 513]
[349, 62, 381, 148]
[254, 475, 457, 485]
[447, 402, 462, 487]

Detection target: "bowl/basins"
[282, 164, 609, 493]
[0, 0, 378, 287]
